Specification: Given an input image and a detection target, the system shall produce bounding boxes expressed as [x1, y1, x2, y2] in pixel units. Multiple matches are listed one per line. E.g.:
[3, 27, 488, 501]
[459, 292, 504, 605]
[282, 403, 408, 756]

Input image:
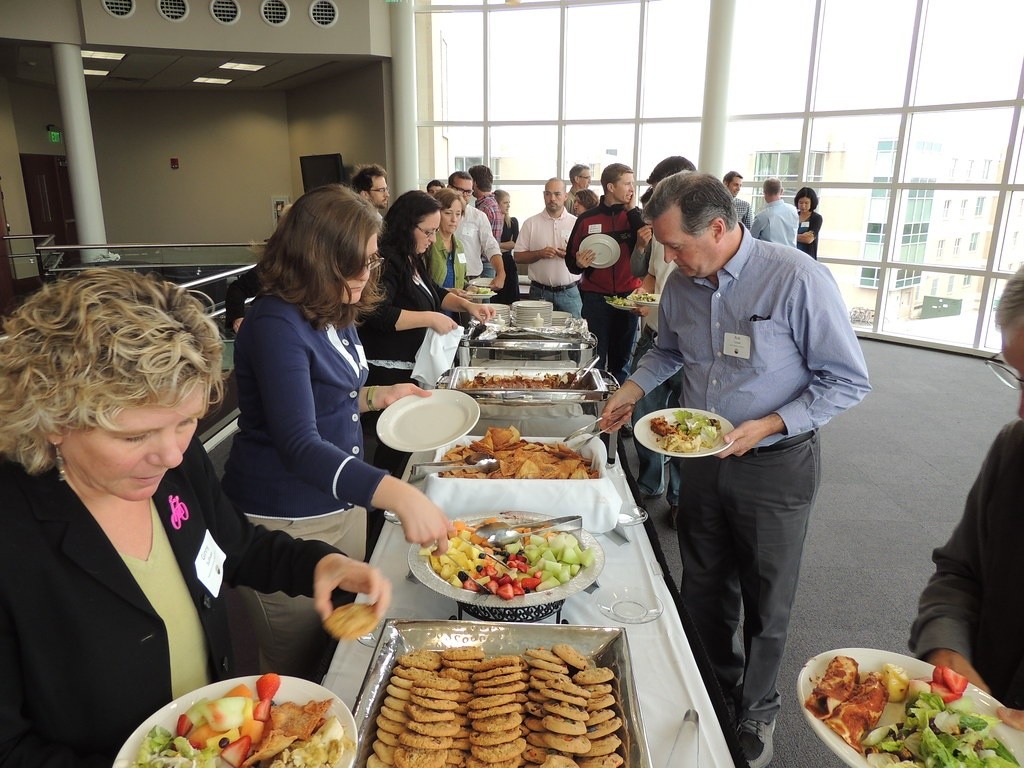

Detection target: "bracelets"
[367, 386, 380, 411]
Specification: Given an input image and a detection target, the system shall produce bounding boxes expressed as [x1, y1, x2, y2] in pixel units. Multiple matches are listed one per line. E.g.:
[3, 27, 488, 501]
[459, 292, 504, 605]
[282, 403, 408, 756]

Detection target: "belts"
[643, 323, 658, 338]
[752, 428, 819, 452]
[531, 281, 576, 292]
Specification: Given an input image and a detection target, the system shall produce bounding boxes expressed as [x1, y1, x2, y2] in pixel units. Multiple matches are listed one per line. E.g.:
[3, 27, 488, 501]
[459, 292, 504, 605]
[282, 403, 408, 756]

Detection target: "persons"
[217, 184, 459, 688]
[0, 268, 389, 768]
[597, 170, 871, 768]
[221, 156, 823, 768]
[906, 264, 1024, 732]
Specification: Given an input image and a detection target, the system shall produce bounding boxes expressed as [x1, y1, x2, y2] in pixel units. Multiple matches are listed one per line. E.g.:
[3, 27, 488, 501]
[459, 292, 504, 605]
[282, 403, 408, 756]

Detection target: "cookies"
[366, 645, 625, 768]
[325, 603, 379, 640]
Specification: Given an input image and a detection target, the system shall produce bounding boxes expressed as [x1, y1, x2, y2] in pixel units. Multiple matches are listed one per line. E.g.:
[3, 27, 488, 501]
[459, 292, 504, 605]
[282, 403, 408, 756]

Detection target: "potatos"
[883, 663, 909, 702]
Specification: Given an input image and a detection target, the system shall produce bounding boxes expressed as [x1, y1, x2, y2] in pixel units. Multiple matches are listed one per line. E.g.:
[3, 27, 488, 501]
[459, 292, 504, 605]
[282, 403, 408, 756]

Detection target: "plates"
[384, 509, 403, 525]
[579, 234, 621, 267]
[626, 294, 661, 306]
[465, 292, 497, 300]
[357, 604, 428, 649]
[471, 300, 573, 328]
[634, 407, 734, 458]
[110, 674, 359, 768]
[467, 278, 495, 286]
[377, 388, 479, 452]
[606, 300, 637, 310]
[597, 587, 664, 625]
[797, 648, 1024, 768]
[616, 504, 648, 526]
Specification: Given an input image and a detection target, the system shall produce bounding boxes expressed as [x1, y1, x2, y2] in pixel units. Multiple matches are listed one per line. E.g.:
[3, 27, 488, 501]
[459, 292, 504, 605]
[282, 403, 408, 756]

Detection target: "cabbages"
[674, 410, 724, 449]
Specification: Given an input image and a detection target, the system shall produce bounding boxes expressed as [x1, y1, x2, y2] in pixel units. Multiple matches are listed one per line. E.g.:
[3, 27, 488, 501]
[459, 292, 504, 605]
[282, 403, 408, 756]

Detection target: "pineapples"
[418, 530, 490, 589]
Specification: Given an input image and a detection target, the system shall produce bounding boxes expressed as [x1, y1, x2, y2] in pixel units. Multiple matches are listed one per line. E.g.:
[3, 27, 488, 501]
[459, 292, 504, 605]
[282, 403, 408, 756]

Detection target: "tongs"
[412, 452, 499, 478]
[667, 709, 700, 768]
[475, 514, 583, 545]
[561, 402, 636, 452]
[557, 355, 600, 390]
[434, 535, 512, 595]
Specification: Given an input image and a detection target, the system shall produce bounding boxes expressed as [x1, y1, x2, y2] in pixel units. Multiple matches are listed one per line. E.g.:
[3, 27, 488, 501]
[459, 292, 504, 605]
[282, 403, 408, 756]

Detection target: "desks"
[323, 322, 746, 768]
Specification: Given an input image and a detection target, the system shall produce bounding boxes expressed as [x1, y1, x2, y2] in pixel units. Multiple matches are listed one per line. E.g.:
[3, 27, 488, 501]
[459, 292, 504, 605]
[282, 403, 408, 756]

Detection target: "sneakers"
[735, 717, 776, 768]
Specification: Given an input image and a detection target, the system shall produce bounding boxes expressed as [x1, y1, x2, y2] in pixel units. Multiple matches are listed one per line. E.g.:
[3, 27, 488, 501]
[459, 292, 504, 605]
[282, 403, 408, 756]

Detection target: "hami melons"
[189, 683, 264, 745]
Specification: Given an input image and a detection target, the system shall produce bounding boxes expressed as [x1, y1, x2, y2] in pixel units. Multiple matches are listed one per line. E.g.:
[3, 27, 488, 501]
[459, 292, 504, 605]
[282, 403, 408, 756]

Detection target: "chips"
[441, 425, 599, 479]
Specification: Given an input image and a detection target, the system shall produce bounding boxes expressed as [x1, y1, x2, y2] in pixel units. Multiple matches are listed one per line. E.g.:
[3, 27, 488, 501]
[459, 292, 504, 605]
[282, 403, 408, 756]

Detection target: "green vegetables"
[125, 725, 226, 768]
[860, 691, 1021, 768]
[603, 291, 654, 306]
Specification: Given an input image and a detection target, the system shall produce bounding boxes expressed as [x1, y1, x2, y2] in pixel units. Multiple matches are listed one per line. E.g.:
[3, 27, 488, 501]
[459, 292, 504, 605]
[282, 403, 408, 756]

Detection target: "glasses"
[984, 352, 1024, 390]
[370, 187, 389, 193]
[578, 176, 591, 179]
[361, 252, 385, 270]
[450, 184, 474, 196]
[416, 225, 440, 238]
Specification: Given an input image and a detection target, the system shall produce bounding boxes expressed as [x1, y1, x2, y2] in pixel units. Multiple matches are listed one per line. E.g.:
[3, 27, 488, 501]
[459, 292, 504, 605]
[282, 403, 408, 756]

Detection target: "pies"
[801, 655, 888, 755]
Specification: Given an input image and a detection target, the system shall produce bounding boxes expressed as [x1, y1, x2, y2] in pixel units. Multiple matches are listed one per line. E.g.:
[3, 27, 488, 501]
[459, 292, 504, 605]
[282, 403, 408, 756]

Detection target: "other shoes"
[642, 492, 663, 500]
[620, 423, 633, 437]
[672, 506, 680, 529]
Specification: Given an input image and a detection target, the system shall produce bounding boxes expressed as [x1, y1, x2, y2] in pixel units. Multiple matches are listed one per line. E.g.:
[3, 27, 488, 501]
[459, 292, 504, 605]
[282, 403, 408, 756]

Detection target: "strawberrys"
[463, 554, 542, 600]
[256, 673, 280, 701]
[176, 714, 193, 737]
[254, 697, 272, 722]
[926, 664, 968, 703]
[220, 735, 252, 768]
